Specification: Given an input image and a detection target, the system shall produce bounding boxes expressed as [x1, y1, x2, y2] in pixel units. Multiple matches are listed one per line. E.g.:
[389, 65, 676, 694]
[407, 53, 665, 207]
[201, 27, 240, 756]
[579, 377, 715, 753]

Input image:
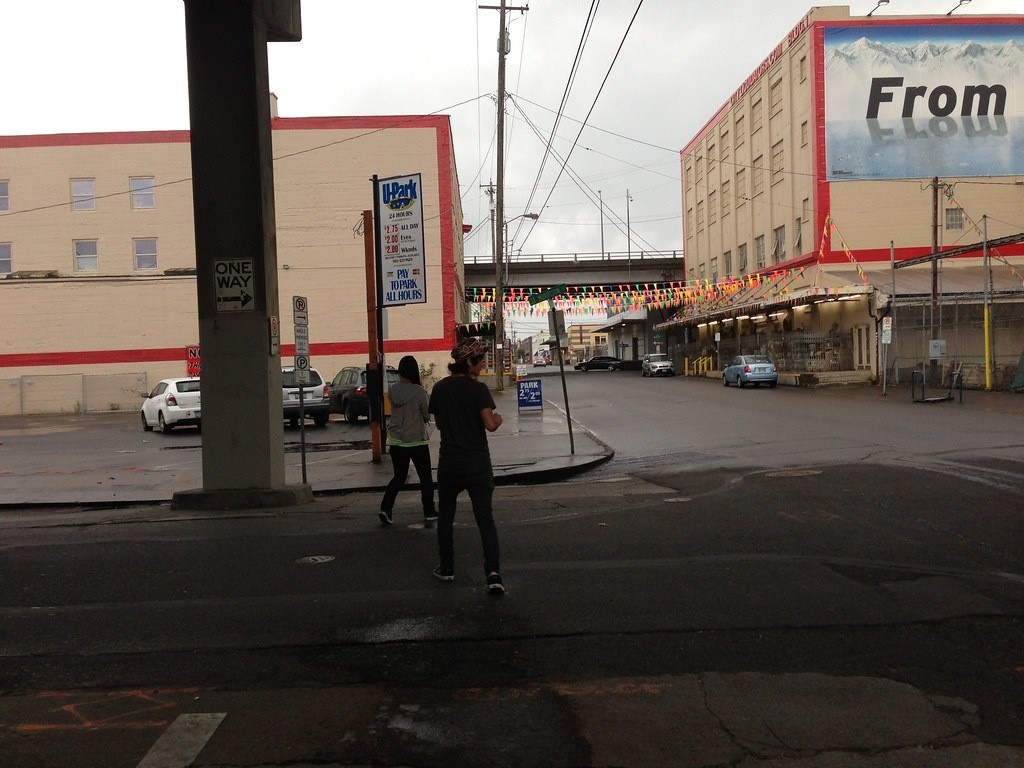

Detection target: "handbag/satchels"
[424, 418, 436, 440]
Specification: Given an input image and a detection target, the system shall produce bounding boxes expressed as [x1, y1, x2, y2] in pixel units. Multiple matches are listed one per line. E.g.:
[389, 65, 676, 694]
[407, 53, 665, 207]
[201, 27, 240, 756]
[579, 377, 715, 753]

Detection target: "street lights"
[495, 213, 539, 394]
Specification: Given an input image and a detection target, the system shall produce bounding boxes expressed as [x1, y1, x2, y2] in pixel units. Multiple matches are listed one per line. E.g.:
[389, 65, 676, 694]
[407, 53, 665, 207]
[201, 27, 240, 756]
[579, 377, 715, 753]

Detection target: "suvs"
[325, 364, 401, 425]
[641, 353, 676, 377]
[281, 365, 332, 427]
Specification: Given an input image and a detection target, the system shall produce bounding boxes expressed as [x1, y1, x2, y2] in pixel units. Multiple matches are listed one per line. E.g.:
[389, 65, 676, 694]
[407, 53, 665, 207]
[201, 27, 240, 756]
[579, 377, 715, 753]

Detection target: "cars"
[532, 357, 546, 367]
[721, 354, 778, 388]
[139, 376, 201, 435]
[574, 355, 622, 372]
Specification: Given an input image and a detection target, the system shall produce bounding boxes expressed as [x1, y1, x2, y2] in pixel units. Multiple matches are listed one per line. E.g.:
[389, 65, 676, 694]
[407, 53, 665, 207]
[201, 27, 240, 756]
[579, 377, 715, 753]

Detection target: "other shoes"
[378, 507, 394, 524]
[423, 509, 438, 520]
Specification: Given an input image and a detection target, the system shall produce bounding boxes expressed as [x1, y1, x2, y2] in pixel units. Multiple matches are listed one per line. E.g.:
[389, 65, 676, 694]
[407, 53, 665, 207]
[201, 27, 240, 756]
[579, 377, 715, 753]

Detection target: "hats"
[451, 337, 490, 360]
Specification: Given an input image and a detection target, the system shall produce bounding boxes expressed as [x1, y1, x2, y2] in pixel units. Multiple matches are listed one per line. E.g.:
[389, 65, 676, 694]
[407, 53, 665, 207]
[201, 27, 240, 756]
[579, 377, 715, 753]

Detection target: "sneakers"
[431, 565, 455, 580]
[486, 574, 505, 592]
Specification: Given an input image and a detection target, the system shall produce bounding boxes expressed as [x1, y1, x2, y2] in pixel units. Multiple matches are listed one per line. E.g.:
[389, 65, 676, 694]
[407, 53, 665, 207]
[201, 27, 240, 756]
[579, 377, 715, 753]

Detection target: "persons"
[428, 338, 505, 592]
[378, 356, 438, 524]
